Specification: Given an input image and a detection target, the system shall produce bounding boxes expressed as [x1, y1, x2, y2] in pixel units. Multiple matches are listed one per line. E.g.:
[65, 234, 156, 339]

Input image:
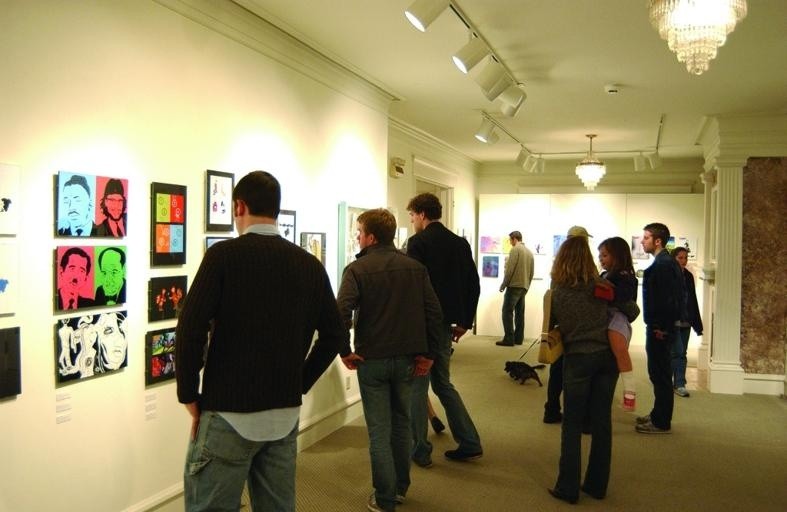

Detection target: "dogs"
[504, 360, 546, 387]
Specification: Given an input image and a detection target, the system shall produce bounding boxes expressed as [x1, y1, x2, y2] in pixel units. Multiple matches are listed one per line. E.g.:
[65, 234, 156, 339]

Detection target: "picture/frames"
[202, 236, 233, 256]
[297, 229, 328, 273]
[276, 208, 298, 245]
[147, 273, 189, 325]
[334, 198, 380, 320]
[202, 168, 237, 234]
[143, 326, 179, 387]
[149, 180, 188, 268]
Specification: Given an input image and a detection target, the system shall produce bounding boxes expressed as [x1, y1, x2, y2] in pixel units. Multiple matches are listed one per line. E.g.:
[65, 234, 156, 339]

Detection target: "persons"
[406, 193, 483, 468]
[174, 170, 342, 512]
[428, 395, 445, 434]
[338, 207, 445, 512]
[496, 230, 535, 346]
[543, 237, 640, 506]
[544, 226, 594, 423]
[58, 176, 130, 381]
[635, 222, 681, 433]
[672, 248, 703, 397]
[598, 237, 638, 410]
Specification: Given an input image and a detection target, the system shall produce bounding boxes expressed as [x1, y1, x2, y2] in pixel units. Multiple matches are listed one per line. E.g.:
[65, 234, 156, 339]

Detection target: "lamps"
[642, 1, 750, 80]
[401, 0, 527, 120]
[466, 118, 546, 175]
[570, 131, 609, 194]
[632, 147, 661, 173]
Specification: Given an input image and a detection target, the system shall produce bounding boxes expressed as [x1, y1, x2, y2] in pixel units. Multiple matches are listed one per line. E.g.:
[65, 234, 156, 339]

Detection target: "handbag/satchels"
[537, 324, 564, 365]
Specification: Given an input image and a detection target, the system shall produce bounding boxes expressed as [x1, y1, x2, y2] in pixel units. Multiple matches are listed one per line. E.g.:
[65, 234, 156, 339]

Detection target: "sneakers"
[431, 416, 446, 434]
[410, 451, 435, 469]
[514, 338, 523, 345]
[672, 385, 690, 397]
[495, 340, 514, 346]
[391, 487, 407, 505]
[547, 487, 579, 505]
[635, 420, 673, 435]
[635, 413, 652, 424]
[579, 484, 607, 500]
[366, 492, 387, 512]
[444, 445, 484, 461]
[623, 391, 637, 410]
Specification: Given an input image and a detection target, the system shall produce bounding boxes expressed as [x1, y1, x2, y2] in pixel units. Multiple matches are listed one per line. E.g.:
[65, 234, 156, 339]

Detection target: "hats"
[567, 226, 593, 239]
